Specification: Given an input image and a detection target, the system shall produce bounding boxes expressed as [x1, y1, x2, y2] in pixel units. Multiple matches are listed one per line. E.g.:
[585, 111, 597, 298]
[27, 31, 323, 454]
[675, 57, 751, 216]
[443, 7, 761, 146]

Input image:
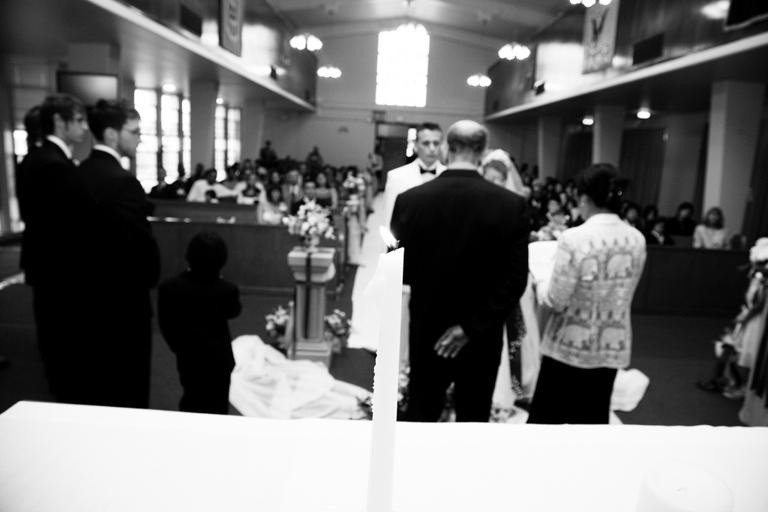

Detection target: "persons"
[384, 122, 446, 381]
[627, 202, 726, 249]
[389, 120, 530, 423]
[526, 163, 648, 423]
[476, 147, 543, 406]
[22, 104, 45, 149]
[522, 163, 584, 227]
[16, 94, 88, 401]
[692, 232, 768, 424]
[152, 139, 382, 225]
[156, 232, 242, 416]
[72, 99, 160, 410]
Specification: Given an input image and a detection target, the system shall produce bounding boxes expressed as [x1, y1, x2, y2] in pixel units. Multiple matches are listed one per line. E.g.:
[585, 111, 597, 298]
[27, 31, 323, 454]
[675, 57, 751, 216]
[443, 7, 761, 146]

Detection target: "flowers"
[262, 301, 352, 355]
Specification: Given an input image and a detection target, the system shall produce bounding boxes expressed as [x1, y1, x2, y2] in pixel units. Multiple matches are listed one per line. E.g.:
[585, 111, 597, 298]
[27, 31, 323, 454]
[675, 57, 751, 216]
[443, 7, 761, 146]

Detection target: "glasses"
[123, 126, 140, 135]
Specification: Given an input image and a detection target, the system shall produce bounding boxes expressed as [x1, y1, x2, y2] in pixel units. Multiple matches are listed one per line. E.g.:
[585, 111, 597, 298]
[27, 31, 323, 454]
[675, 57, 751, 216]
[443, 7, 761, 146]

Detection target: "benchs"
[135, 184, 351, 296]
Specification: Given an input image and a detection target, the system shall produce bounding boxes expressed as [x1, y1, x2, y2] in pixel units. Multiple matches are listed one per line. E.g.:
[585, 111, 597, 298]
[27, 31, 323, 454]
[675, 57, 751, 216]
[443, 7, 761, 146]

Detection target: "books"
[527, 239, 560, 278]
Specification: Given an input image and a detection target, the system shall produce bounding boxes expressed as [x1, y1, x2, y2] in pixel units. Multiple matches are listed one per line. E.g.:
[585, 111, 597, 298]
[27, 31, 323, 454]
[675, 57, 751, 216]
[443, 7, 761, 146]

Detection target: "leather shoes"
[696, 377, 725, 390]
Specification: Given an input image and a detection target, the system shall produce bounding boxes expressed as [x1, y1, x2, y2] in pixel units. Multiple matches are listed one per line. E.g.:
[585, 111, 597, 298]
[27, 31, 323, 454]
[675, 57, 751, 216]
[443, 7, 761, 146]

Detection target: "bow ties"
[420, 167, 436, 174]
